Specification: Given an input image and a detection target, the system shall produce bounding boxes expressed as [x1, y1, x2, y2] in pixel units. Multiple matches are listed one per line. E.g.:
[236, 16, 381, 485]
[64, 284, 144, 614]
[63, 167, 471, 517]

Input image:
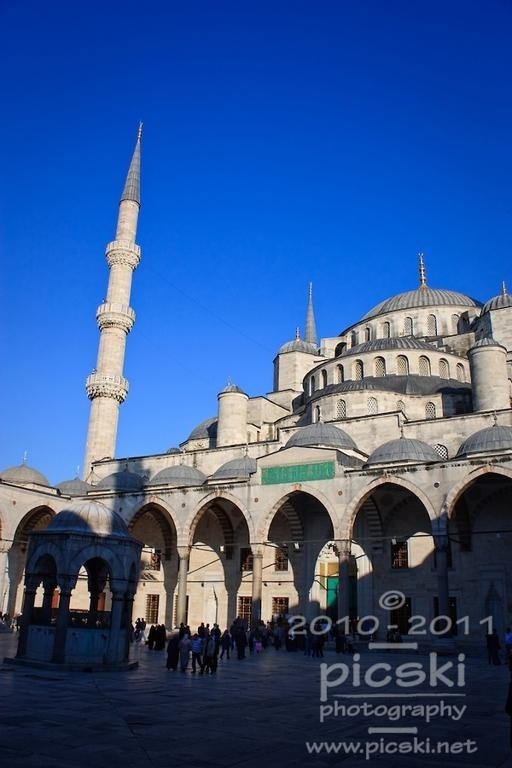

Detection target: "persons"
[485, 625, 511, 670]
[0, 607, 23, 632]
[129, 614, 404, 676]
